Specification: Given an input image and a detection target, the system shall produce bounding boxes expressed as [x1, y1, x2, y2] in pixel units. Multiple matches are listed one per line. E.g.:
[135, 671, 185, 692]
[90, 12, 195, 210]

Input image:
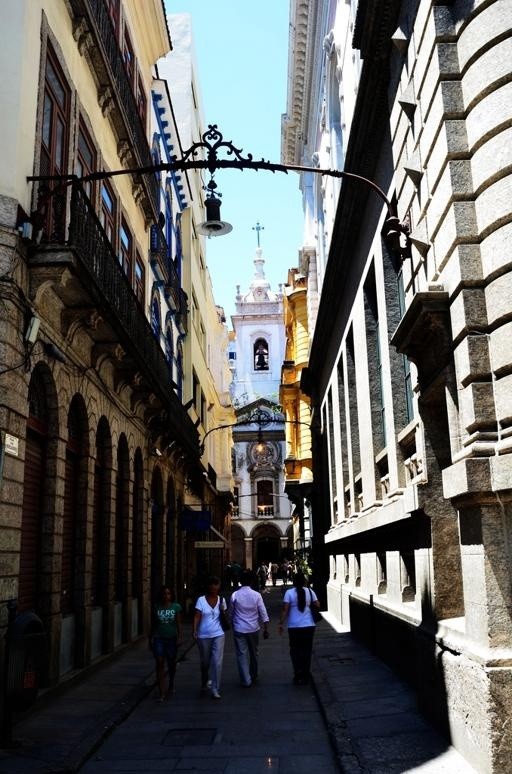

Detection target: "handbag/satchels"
[218, 610, 231, 630]
[309, 602, 324, 624]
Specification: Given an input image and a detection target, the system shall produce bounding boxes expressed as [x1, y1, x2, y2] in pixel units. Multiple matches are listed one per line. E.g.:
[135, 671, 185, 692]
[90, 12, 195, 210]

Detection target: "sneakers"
[242, 675, 260, 686]
[202, 679, 222, 698]
[154, 683, 176, 702]
[291, 672, 312, 684]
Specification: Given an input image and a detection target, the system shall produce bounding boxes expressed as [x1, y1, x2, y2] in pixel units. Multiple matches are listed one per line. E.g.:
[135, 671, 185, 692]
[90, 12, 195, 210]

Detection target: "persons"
[227, 559, 292, 592]
[277, 572, 320, 685]
[147, 585, 183, 703]
[228, 573, 270, 688]
[192, 576, 227, 699]
